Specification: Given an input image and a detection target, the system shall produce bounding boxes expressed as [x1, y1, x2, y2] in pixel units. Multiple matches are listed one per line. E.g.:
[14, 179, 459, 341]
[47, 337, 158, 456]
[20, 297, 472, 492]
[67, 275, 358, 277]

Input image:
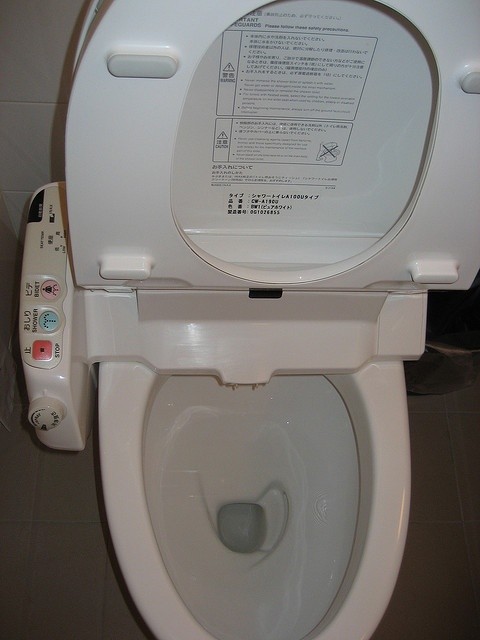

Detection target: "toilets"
[19, 0, 479, 640]
